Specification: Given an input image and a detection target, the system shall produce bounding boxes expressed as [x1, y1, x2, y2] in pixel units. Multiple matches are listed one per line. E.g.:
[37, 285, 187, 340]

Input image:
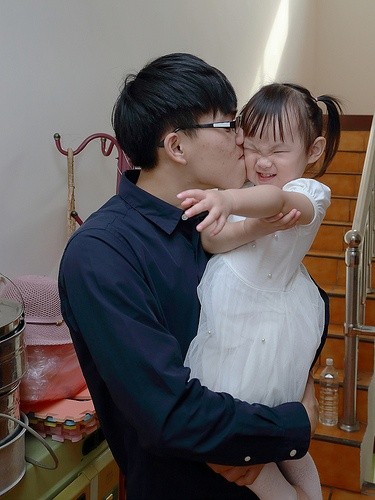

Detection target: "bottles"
[318, 358, 339, 427]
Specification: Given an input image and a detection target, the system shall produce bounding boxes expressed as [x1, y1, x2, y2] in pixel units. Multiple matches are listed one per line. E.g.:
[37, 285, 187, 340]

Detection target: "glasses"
[157, 115, 241, 147]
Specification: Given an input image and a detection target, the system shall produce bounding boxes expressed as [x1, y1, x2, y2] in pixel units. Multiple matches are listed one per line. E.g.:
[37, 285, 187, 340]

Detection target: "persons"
[58, 54, 318, 500]
[175, 84, 344, 500]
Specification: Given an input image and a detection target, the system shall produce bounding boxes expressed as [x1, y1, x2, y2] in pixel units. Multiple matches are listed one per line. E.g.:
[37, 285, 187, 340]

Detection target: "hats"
[0, 276, 73, 345]
